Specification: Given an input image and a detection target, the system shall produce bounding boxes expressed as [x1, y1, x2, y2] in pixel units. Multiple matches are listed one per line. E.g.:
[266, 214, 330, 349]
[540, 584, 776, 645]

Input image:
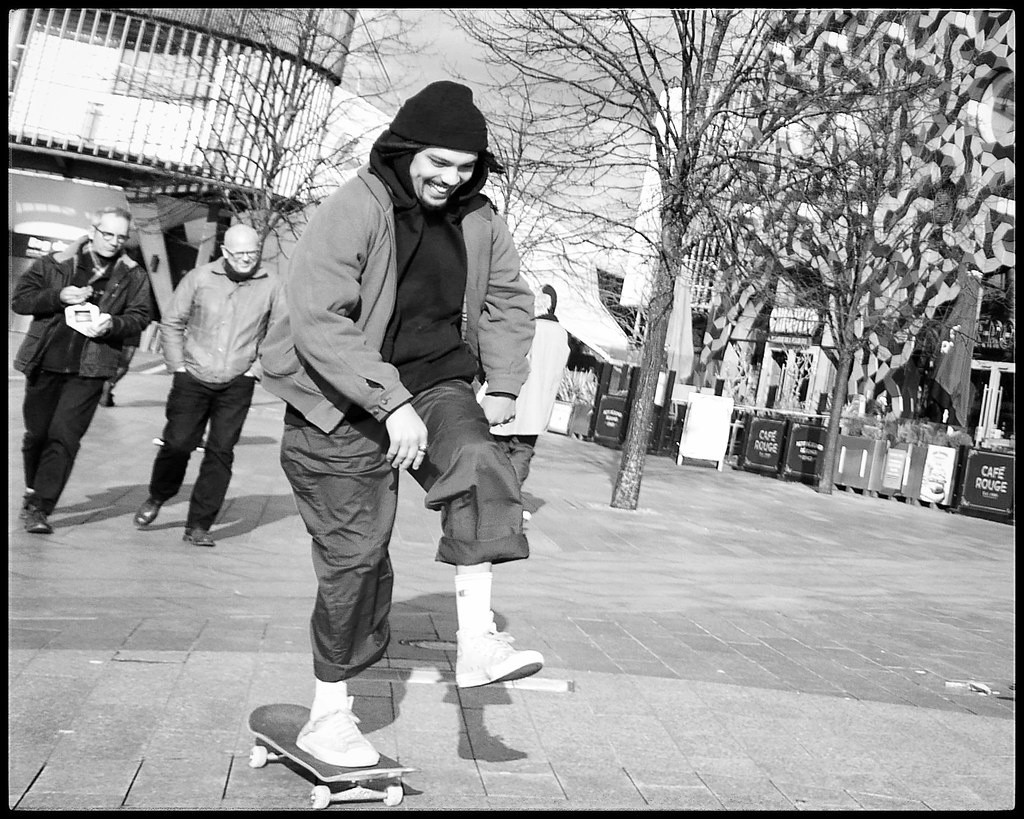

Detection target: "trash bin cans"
[834, 432, 887, 494]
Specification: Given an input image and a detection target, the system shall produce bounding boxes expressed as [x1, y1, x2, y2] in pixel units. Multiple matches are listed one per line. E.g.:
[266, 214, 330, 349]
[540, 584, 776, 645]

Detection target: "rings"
[418, 446, 428, 452]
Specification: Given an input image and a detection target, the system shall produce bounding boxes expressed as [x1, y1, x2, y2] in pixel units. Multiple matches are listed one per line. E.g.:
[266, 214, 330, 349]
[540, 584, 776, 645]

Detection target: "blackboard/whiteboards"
[677, 393, 734, 461]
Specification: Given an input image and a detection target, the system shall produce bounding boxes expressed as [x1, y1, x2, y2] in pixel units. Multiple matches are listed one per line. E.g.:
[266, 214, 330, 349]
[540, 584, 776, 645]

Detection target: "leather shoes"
[133, 496, 164, 525]
[183, 525, 215, 546]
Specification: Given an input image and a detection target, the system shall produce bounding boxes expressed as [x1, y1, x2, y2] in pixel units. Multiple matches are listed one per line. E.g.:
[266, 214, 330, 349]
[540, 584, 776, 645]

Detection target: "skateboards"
[248, 704, 420, 810]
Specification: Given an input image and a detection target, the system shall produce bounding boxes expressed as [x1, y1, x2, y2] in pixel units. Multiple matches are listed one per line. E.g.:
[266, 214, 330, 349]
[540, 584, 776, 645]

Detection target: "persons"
[259, 82, 548, 768]
[135, 224, 281, 546]
[478, 286, 571, 491]
[9, 206, 152, 534]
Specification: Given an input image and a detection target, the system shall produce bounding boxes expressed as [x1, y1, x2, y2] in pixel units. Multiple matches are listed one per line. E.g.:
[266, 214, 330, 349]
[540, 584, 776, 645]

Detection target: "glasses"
[225, 248, 261, 259]
[93, 225, 130, 244]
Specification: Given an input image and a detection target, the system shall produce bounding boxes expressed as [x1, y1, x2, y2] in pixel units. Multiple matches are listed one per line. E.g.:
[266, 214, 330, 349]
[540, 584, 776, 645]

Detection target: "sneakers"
[298, 695, 379, 768]
[19, 492, 34, 519]
[24, 505, 51, 532]
[455, 613, 543, 689]
